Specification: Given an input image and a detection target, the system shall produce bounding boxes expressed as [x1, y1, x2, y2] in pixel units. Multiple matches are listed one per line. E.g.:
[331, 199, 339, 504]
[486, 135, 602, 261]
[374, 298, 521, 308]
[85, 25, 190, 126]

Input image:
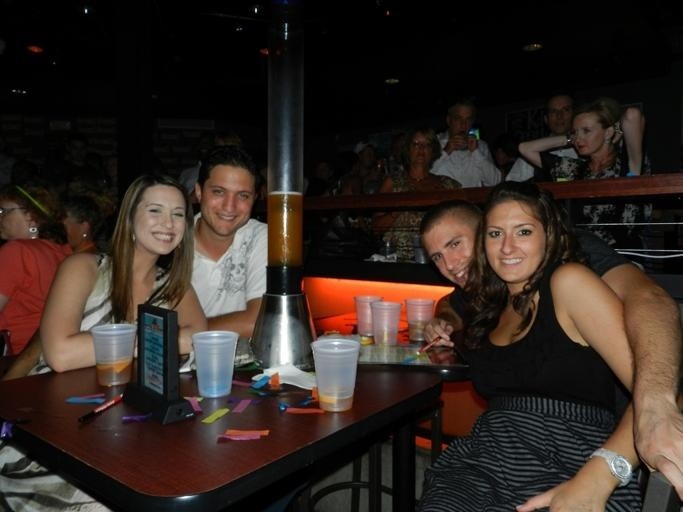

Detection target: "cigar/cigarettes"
[415, 335, 443, 357]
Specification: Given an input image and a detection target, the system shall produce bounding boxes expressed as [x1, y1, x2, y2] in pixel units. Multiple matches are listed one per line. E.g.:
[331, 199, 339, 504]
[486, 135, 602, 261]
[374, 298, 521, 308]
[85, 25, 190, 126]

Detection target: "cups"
[312, 338, 359, 412]
[351, 294, 384, 335]
[405, 300, 433, 342]
[192, 330, 239, 397]
[367, 302, 401, 344]
[93, 325, 137, 385]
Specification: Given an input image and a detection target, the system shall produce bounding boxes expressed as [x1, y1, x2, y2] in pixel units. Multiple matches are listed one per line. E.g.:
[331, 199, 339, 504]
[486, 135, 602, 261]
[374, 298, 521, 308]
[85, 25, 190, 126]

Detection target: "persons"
[1, 181, 119, 370]
[420, 190, 638, 511]
[423, 199, 682, 511]
[188, 158, 271, 343]
[2, 133, 268, 237]
[37, 173, 209, 374]
[303, 90, 653, 261]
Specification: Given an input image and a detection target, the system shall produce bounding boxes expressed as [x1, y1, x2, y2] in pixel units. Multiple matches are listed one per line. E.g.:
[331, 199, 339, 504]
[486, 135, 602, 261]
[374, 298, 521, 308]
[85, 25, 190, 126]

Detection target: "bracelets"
[565, 131, 572, 148]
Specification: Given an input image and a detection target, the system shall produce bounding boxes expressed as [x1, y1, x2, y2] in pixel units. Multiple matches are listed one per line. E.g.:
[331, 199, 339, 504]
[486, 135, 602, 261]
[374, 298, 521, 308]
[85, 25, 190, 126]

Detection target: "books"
[177, 336, 258, 373]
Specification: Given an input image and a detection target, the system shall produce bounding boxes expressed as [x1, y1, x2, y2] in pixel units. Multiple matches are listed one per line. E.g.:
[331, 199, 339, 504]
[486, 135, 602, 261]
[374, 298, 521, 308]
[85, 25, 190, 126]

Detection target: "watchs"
[586, 440, 634, 491]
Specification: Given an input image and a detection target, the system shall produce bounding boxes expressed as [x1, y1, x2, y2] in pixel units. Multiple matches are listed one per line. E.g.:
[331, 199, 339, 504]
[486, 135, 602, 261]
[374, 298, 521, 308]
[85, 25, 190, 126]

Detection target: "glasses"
[0, 207, 25, 216]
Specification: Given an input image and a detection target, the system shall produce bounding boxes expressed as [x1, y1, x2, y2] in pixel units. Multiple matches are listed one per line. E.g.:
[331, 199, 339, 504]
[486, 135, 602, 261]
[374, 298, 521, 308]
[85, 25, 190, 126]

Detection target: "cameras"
[463, 126, 480, 145]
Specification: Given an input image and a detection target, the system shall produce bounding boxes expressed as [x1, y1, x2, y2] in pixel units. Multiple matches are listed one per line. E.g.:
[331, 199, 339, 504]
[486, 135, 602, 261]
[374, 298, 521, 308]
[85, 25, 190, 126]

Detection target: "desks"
[0, 361, 445, 512]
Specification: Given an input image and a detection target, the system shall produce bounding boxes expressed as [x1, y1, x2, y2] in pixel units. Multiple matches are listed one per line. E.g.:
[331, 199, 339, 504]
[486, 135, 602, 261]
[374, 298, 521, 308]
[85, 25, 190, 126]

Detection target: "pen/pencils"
[415, 336, 442, 355]
[77, 393, 124, 424]
